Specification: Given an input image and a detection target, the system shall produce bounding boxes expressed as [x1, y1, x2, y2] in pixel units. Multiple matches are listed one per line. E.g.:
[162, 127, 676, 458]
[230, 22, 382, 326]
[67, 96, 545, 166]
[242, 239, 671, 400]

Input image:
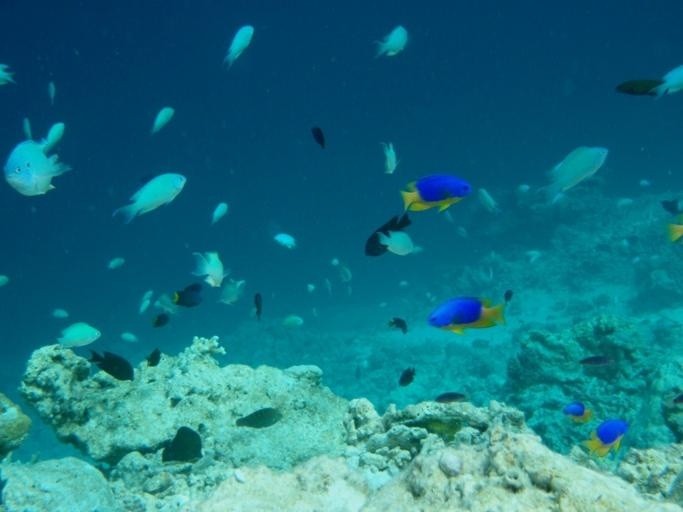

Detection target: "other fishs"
[661, 195, 683, 217]
[561, 394, 590, 428]
[474, 144, 652, 263]
[427, 291, 513, 336]
[435, 391, 465, 403]
[387, 317, 409, 334]
[579, 354, 611, 366]
[666, 222, 682, 244]
[398, 367, 416, 387]
[398, 171, 472, 216]
[583, 413, 636, 454]
[43, 201, 354, 383]
[379, 142, 400, 179]
[375, 227, 422, 260]
[365, 216, 412, 266]
